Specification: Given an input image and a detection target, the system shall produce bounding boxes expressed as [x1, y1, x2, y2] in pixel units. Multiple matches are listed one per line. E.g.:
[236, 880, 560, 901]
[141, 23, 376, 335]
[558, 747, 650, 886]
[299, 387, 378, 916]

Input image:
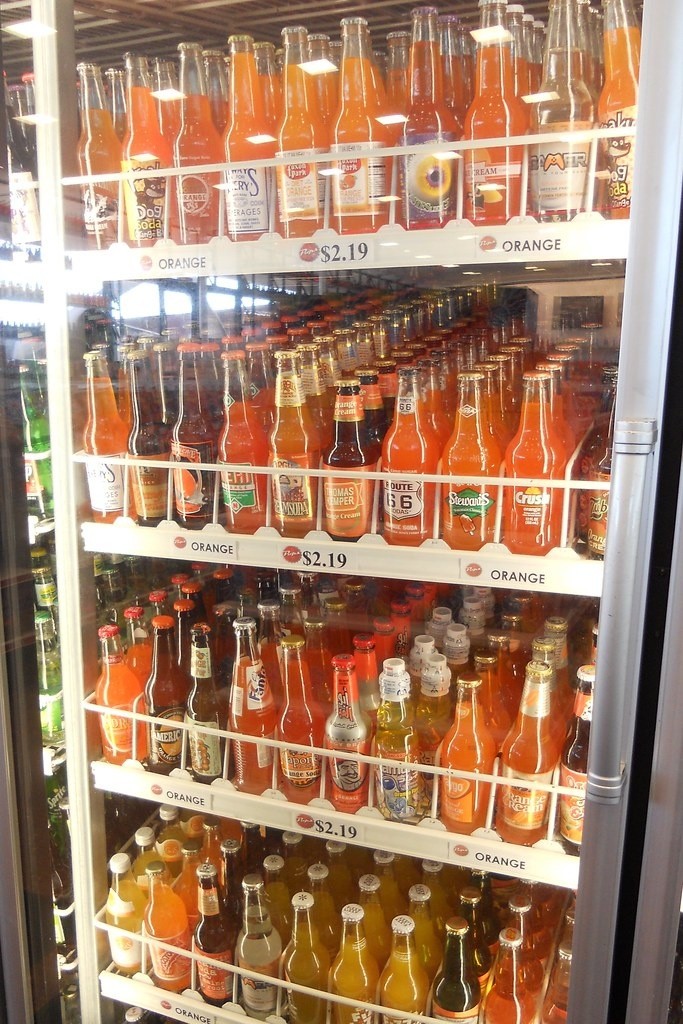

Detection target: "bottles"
[0, 0, 642, 1024]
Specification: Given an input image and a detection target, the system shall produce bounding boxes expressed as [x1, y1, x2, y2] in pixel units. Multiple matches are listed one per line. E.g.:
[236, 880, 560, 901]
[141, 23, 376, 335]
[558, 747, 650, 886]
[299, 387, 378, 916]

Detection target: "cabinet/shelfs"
[0, 0, 683, 1024]
